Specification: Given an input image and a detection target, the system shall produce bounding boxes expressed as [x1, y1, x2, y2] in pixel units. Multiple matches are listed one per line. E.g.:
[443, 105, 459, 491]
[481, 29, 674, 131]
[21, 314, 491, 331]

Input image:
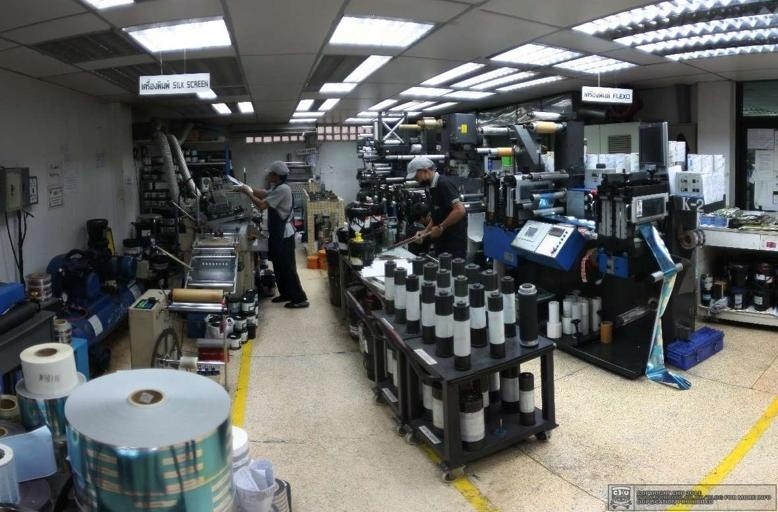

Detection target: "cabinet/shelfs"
[696, 227, 778, 327]
[138, 142, 231, 233]
[339, 255, 560, 483]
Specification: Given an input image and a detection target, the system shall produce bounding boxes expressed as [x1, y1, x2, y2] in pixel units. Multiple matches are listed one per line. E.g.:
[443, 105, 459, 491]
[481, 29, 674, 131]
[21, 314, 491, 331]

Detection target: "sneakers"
[272, 295, 289, 303]
[284, 298, 309, 308]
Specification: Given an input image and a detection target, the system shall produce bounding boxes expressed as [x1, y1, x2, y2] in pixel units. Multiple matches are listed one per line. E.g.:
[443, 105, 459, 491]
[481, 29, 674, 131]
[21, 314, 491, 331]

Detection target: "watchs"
[438, 224, 445, 230]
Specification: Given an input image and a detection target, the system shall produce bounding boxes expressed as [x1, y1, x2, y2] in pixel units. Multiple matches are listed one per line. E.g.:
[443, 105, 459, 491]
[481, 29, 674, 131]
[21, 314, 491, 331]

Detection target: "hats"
[265, 160, 290, 176]
[404, 156, 433, 179]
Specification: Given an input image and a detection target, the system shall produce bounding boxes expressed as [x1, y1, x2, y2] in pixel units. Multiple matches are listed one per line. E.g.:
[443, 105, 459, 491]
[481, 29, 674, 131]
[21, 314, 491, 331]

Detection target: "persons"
[405, 156, 468, 258]
[239, 161, 310, 308]
[404, 201, 433, 257]
